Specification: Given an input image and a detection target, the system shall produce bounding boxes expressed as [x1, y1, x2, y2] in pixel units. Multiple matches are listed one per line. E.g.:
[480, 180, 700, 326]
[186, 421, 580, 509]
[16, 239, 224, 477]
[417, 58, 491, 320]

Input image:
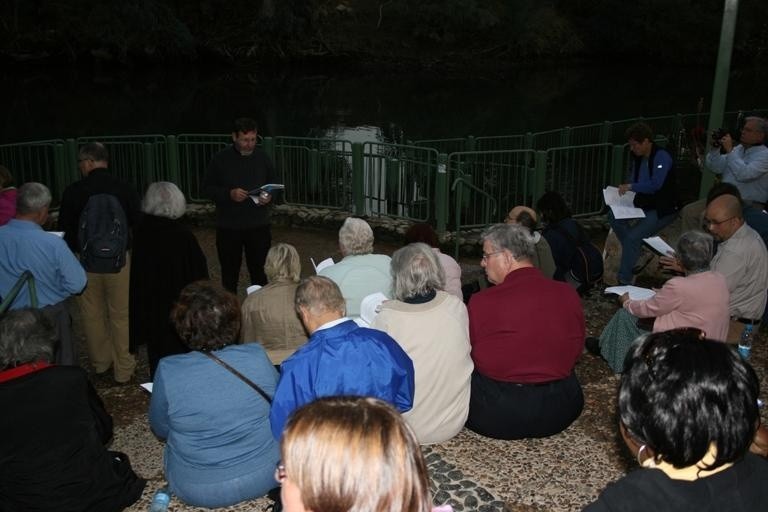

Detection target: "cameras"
[712, 128, 728, 140]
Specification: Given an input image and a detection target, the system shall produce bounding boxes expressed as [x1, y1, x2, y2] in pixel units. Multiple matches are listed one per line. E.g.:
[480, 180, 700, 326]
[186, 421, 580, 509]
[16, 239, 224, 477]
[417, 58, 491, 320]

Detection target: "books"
[248, 182, 285, 197]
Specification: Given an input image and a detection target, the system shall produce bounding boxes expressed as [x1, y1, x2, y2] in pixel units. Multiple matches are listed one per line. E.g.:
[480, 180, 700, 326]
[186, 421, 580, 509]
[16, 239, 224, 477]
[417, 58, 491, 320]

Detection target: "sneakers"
[584, 336, 604, 358]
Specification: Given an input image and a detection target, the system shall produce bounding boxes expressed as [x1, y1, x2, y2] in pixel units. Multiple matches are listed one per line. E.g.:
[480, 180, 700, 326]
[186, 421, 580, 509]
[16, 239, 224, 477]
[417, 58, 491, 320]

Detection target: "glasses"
[705, 218, 734, 230]
[481, 251, 502, 260]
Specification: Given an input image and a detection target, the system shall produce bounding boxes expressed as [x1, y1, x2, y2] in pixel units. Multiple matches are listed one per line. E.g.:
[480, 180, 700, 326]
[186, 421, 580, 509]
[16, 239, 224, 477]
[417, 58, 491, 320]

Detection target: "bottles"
[737, 324, 754, 360]
[150, 487, 172, 512]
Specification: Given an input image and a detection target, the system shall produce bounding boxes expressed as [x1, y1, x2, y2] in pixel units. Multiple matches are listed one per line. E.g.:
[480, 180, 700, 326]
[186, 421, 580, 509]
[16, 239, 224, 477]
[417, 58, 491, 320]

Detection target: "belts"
[731, 314, 761, 326]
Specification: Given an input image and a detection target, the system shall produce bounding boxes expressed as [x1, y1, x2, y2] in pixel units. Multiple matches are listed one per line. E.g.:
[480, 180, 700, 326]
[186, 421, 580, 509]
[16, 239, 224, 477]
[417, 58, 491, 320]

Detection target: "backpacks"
[74, 172, 127, 274]
[557, 222, 604, 288]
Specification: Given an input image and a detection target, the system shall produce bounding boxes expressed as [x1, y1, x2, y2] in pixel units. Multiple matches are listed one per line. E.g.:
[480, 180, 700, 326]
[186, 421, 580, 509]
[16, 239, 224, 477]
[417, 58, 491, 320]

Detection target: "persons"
[144, 277, 278, 509]
[271, 395, 430, 511]
[205, 116, 277, 291]
[0, 182, 89, 365]
[0, 304, 146, 512]
[129, 180, 210, 381]
[575, 328, 767, 512]
[56, 140, 139, 385]
[239, 112, 768, 449]
[0, 162, 19, 226]
